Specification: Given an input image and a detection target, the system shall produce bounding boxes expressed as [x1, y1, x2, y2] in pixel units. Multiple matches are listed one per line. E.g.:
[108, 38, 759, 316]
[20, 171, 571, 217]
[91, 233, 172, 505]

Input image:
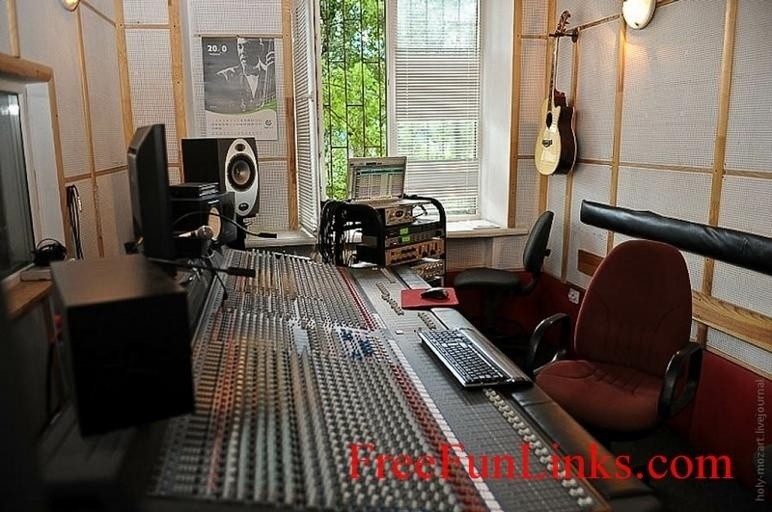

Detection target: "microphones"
[192, 266, 256, 278]
[206, 256, 228, 300]
[210, 213, 277, 238]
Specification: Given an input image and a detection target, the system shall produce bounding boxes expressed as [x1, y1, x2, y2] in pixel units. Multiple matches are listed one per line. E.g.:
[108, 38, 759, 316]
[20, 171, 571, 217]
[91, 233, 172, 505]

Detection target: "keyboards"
[417, 327, 534, 389]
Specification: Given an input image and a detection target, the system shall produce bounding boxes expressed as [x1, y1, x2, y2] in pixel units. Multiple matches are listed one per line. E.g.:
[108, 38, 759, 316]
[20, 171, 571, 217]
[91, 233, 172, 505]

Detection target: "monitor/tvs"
[127, 124, 172, 252]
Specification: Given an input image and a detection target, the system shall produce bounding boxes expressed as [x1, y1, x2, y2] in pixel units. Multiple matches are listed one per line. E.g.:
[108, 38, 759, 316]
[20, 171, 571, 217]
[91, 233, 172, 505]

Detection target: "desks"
[243, 219, 527, 274]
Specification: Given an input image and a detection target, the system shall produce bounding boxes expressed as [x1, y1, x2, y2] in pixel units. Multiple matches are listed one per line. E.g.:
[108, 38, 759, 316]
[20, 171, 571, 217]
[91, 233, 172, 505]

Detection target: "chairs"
[453, 211, 553, 342]
[524, 239, 701, 472]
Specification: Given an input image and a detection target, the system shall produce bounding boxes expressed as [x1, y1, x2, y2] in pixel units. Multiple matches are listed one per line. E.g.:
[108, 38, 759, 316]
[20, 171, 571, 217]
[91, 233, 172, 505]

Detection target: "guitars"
[535, 10, 577, 178]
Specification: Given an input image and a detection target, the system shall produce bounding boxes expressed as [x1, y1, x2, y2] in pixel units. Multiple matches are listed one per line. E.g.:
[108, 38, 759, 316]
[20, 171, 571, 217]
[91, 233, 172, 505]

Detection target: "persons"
[212, 38, 267, 116]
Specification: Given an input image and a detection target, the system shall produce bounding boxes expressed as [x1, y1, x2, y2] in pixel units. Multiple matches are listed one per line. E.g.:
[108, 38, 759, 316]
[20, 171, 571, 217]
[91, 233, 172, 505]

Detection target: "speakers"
[50, 253, 195, 439]
[181, 137, 260, 216]
[171, 192, 237, 244]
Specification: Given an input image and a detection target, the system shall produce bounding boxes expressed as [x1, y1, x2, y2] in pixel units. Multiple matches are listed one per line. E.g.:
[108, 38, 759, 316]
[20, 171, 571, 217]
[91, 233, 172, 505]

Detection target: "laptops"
[346, 157, 431, 209]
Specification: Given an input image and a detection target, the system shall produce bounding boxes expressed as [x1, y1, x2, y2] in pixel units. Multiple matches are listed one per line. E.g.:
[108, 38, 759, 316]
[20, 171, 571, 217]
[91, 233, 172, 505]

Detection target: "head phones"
[34, 238, 67, 266]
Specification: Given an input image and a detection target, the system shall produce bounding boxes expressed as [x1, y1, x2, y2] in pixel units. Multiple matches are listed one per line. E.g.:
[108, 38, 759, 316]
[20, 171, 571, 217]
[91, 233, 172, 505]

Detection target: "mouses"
[421, 288, 449, 300]
